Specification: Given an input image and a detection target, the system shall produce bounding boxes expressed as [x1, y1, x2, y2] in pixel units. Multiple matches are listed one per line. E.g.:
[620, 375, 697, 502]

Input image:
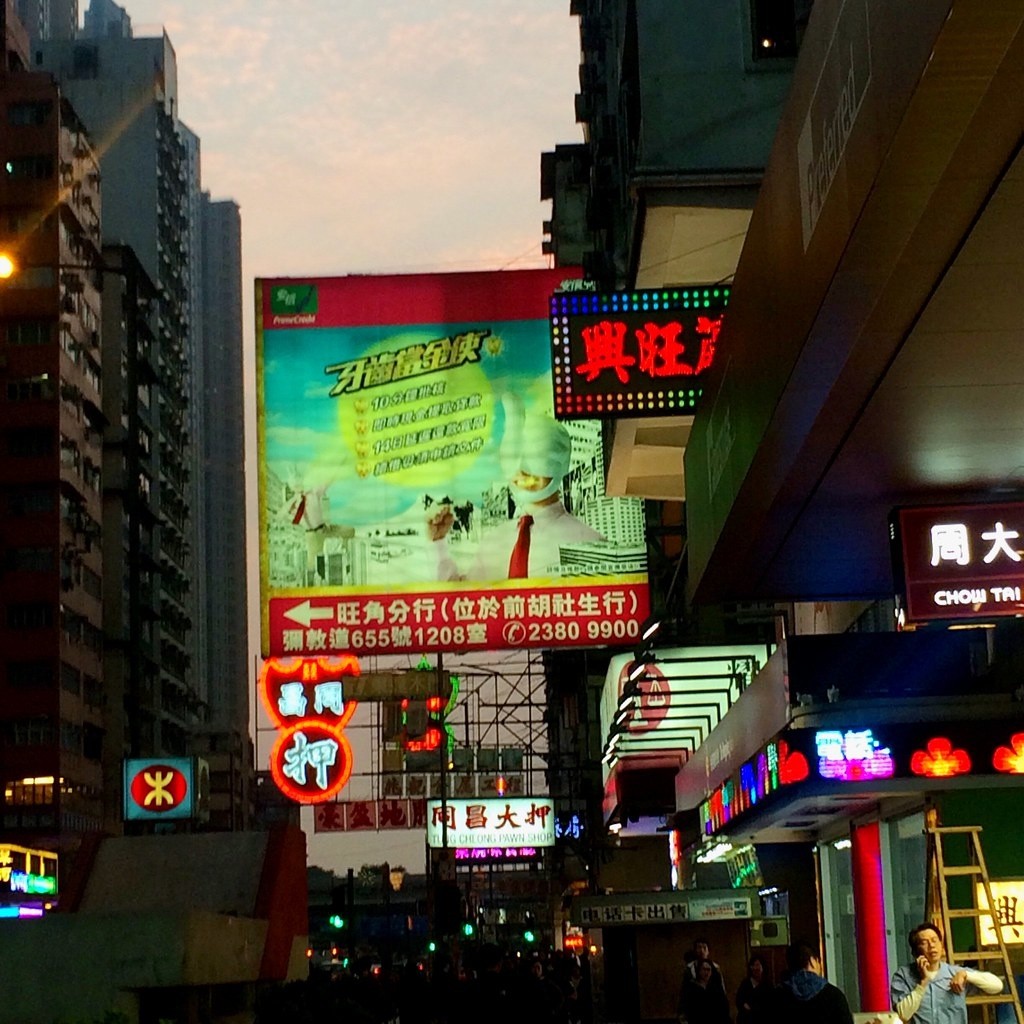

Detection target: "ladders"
[923, 827, 1024, 1024]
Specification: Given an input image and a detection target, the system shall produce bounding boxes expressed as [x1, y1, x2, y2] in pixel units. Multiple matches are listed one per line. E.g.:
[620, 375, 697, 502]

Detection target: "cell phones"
[912, 948, 923, 958]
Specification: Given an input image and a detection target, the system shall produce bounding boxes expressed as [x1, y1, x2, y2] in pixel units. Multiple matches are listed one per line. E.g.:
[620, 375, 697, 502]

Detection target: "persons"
[331, 942, 592, 1024]
[776, 942, 853, 1024]
[273, 452, 350, 586]
[736, 954, 773, 1024]
[677, 940, 732, 1024]
[422, 416, 606, 582]
[890, 922, 1003, 1024]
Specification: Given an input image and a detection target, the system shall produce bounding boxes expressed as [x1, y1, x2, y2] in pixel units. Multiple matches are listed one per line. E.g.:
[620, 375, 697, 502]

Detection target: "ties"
[507, 515, 534, 579]
[292, 495, 307, 524]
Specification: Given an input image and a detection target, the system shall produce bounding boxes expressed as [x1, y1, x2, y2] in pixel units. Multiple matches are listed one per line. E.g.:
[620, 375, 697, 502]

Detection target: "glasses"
[701, 967, 711, 971]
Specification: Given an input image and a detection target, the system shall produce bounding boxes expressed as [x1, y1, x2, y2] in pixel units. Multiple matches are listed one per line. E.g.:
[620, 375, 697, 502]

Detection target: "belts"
[305, 523, 325, 532]
